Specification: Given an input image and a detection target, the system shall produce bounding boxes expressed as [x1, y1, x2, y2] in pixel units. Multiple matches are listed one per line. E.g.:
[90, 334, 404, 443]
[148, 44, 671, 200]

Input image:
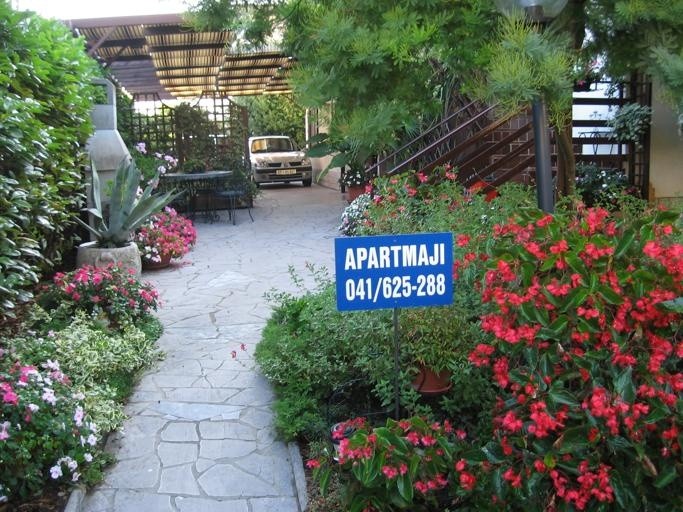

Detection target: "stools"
[210, 191, 255, 225]
[193, 189, 215, 218]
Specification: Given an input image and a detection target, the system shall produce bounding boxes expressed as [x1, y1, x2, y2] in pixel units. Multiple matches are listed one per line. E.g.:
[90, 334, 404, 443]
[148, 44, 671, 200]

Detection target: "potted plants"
[39, 152, 188, 282]
[337, 161, 369, 204]
[402, 305, 456, 398]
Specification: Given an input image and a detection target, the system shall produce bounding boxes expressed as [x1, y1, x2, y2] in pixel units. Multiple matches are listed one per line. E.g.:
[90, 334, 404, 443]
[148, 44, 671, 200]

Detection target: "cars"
[246, 135, 312, 188]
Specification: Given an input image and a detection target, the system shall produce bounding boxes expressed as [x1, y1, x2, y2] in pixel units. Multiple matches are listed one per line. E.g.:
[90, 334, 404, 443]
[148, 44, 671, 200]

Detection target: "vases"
[141, 254, 172, 271]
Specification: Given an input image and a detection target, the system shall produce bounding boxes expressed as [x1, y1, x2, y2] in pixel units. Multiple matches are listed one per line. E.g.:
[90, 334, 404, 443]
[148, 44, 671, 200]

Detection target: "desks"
[162, 170, 234, 225]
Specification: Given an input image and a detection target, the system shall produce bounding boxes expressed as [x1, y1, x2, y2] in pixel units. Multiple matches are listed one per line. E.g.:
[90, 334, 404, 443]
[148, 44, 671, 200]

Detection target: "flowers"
[128, 206, 196, 262]
